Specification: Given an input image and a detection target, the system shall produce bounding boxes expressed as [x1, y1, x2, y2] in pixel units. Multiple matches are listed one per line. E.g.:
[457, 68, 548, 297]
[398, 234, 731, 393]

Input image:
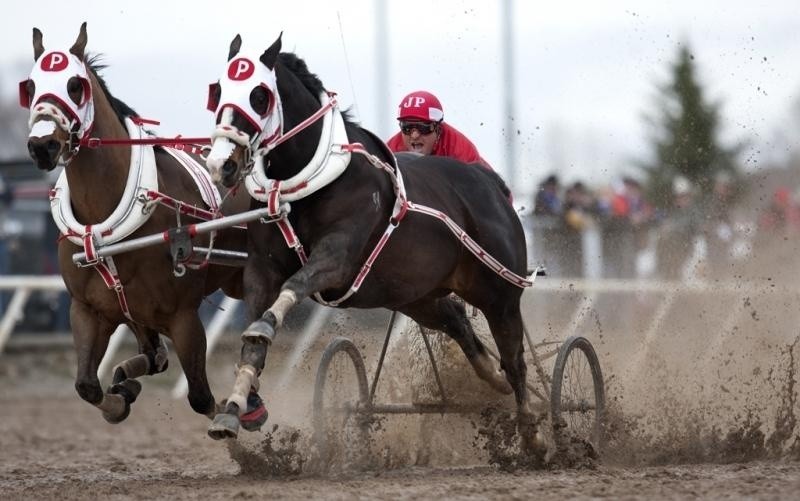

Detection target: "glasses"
[399, 120, 435, 135]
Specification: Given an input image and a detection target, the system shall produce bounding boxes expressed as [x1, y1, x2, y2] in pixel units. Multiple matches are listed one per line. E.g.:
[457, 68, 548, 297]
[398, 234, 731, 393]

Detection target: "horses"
[206, 31, 549, 458]
[19, 21, 264, 423]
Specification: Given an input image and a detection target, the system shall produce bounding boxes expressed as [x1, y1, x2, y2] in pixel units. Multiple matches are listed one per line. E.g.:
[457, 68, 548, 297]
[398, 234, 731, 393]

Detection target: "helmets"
[397, 91, 444, 124]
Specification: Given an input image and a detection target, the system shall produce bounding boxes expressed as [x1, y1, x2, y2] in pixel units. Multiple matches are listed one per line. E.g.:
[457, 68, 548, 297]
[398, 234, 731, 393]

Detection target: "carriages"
[19, 21, 607, 465]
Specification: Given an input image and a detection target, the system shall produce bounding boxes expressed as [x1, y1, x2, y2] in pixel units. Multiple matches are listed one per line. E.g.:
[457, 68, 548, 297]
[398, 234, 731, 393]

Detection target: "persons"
[533, 166, 800, 282]
[386, 92, 512, 206]
